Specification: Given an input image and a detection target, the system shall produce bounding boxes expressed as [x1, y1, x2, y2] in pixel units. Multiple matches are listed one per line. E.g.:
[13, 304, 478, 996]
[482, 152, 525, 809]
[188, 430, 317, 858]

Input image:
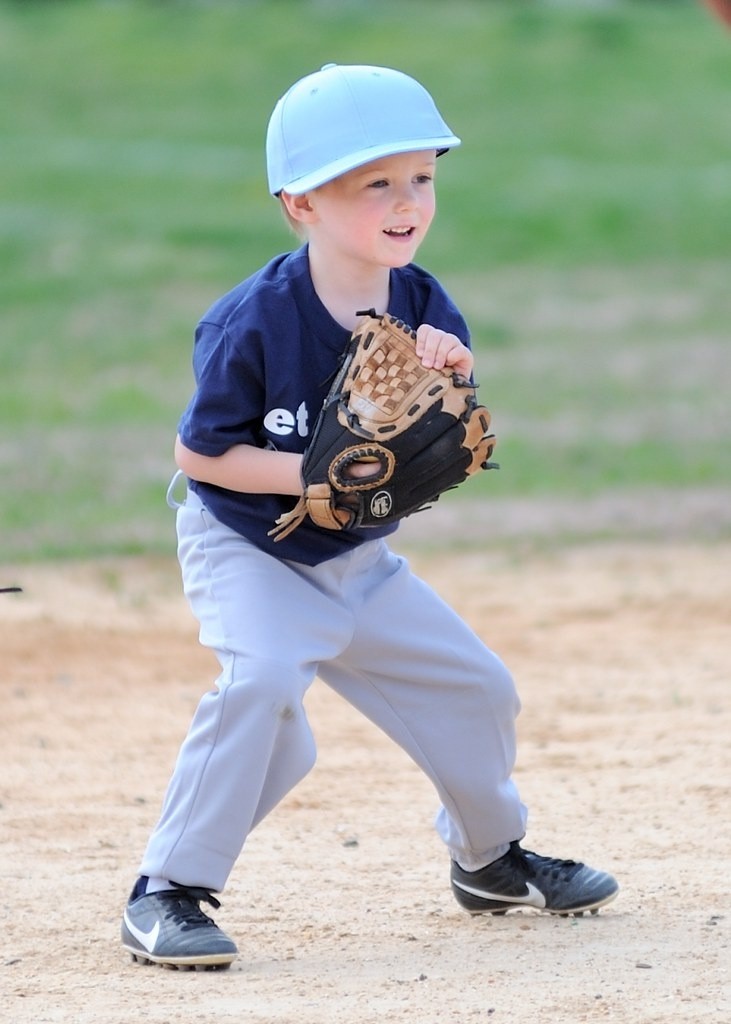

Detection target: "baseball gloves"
[296, 307, 498, 533]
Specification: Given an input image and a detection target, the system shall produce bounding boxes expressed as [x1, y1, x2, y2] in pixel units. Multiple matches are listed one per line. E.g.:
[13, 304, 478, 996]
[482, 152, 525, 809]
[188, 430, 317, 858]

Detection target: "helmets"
[266, 63, 460, 198]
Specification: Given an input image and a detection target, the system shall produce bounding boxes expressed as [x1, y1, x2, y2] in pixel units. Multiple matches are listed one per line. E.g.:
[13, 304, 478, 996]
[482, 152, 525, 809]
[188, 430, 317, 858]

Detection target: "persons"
[119, 64, 620, 973]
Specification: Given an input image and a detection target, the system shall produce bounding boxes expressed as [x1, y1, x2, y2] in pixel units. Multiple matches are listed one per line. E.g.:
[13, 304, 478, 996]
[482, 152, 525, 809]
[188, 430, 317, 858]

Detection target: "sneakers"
[120, 875, 240, 970]
[449, 845, 619, 916]
